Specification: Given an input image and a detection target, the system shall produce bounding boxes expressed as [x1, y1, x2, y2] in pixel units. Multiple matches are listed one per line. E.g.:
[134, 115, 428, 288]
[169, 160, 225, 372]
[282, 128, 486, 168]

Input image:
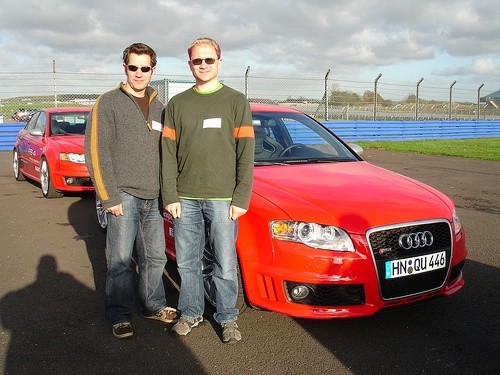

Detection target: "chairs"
[235, 126, 285, 159]
[74, 124, 85, 132]
[57, 122, 71, 132]
[50, 120, 59, 134]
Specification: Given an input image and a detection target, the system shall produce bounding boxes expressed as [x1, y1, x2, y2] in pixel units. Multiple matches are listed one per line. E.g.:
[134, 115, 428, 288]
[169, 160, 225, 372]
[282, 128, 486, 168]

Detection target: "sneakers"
[141, 306, 181, 323]
[170, 315, 203, 336]
[221, 322, 241, 344]
[113, 322, 134, 339]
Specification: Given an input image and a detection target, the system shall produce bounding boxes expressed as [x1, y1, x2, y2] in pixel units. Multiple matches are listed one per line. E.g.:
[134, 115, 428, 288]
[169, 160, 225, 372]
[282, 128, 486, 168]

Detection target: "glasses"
[192, 58, 215, 65]
[125, 65, 156, 72]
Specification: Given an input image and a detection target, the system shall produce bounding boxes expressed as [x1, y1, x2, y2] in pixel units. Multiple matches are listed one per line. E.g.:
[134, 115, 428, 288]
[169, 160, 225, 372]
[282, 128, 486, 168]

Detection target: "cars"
[95, 102, 468, 319]
[12, 107, 95, 198]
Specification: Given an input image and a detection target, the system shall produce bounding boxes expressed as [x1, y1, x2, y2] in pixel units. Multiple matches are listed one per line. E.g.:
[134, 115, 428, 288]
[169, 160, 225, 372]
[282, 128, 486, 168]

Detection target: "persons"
[85, 43, 181, 339]
[160, 37, 255, 344]
